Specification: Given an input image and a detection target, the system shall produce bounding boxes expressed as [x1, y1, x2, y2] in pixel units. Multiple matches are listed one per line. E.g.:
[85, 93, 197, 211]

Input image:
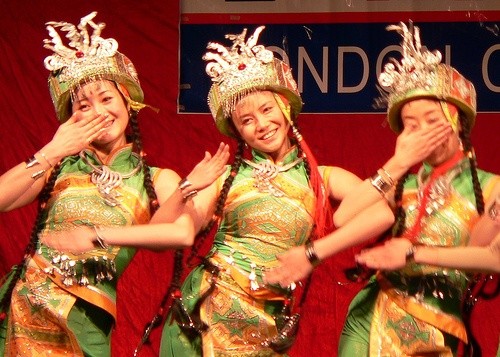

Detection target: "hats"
[379, 19, 477, 133]
[202, 25, 301, 137]
[43, 11, 160, 123]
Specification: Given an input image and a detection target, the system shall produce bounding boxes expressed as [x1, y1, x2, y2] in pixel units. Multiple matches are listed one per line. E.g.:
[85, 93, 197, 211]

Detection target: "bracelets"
[24, 150, 52, 180]
[304, 240, 324, 267]
[177, 178, 197, 202]
[366, 165, 395, 195]
[87, 224, 109, 250]
[405, 243, 416, 265]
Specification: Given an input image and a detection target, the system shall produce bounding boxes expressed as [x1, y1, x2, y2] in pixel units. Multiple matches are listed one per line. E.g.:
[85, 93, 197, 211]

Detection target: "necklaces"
[409, 165, 465, 214]
[242, 156, 302, 197]
[79, 150, 143, 206]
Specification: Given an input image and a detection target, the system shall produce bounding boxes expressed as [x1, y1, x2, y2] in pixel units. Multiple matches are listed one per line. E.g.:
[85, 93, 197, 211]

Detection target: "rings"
[87, 138, 92, 144]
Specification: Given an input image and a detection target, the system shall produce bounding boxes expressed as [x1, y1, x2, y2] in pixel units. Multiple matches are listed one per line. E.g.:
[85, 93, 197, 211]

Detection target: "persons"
[0, 11, 196, 357]
[145, 25, 395, 357]
[333, 19, 500, 357]
